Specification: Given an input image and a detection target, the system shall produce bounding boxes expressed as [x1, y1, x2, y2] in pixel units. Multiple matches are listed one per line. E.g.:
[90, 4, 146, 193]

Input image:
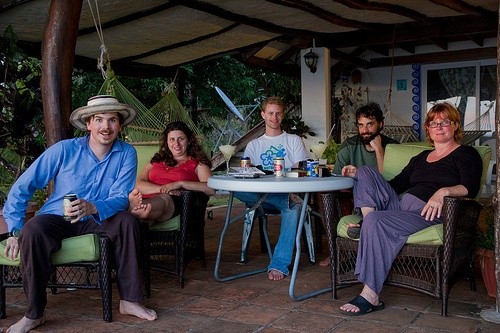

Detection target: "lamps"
[303, 48, 319, 73]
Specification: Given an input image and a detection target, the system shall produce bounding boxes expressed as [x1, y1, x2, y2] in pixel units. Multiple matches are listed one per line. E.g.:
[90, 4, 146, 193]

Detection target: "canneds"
[63, 194, 77, 222]
[240, 157, 250, 167]
[273, 157, 286, 178]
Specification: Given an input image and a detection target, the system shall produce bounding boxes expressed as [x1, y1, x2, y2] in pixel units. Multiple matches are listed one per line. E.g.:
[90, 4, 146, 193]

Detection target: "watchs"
[9, 228, 20, 237]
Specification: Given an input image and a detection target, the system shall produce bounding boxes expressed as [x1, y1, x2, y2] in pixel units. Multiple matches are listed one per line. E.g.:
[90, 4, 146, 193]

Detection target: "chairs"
[0, 175, 117, 324]
[130, 142, 207, 288]
[336, 142, 494, 316]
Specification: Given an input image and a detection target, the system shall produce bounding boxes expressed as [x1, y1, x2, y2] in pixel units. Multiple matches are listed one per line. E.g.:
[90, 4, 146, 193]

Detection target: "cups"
[307, 160, 319, 177]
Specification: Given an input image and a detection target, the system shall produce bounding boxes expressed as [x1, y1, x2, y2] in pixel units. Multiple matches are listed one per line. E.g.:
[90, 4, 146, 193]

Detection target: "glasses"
[426, 120, 456, 128]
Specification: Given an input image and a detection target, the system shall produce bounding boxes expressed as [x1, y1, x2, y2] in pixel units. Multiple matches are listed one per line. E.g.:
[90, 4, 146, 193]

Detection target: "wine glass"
[220, 147, 237, 176]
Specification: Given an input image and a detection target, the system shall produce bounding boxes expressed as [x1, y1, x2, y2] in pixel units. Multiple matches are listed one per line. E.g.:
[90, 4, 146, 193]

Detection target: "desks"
[206, 171, 355, 300]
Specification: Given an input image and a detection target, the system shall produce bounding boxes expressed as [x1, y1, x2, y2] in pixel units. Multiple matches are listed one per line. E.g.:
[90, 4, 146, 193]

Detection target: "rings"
[430, 205, 434, 209]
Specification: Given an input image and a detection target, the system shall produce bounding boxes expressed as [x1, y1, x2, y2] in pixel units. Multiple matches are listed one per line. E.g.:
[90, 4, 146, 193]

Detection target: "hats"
[69, 95, 137, 133]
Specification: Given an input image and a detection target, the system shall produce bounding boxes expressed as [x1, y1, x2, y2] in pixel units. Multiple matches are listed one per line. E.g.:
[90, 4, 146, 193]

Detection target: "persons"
[337, 102, 483, 316]
[2, 95, 214, 333]
[229, 97, 308, 282]
[319, 102, 401, 270]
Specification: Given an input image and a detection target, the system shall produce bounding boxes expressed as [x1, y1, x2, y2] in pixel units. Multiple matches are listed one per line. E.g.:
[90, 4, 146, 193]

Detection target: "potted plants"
[0, 147, 38, 234]
[321, 137, 341, 172]
[471, 205, 496, 298]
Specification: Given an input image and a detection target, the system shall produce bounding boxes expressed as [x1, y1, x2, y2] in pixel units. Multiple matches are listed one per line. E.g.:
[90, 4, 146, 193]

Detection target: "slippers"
[347, 219, 363, 240]
[338, 294, 385, 316]
